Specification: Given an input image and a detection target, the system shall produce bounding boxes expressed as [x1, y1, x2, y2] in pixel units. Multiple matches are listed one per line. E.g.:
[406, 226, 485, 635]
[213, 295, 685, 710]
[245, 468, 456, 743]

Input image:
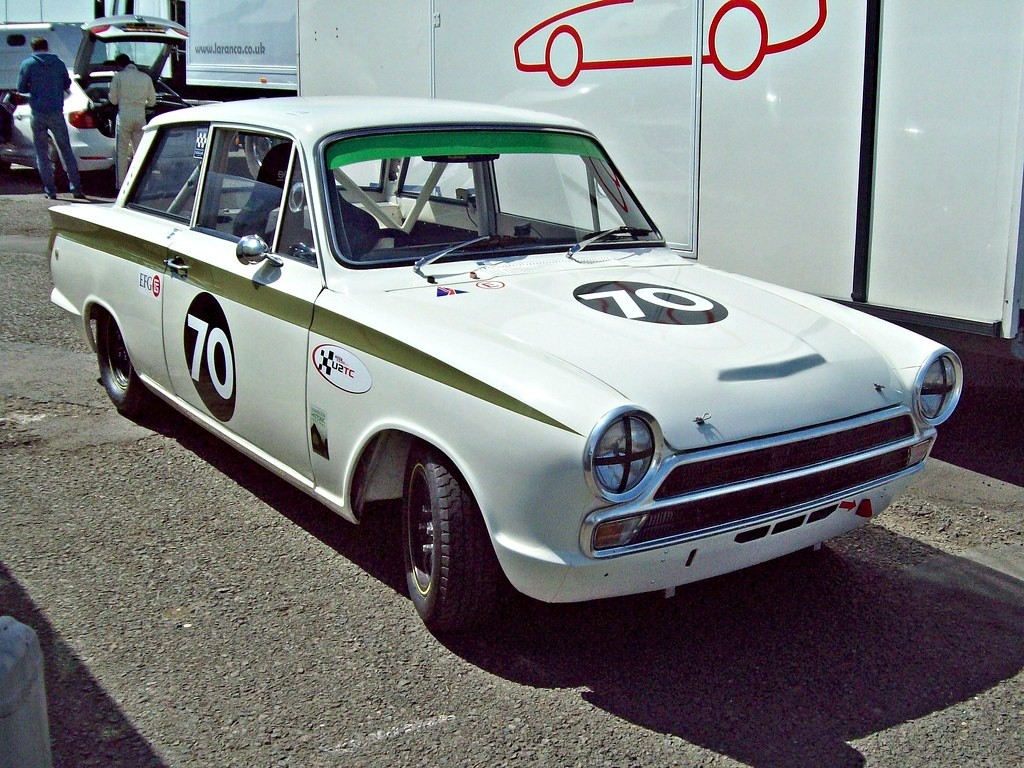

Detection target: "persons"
[16, 37, 87, 199]
[108, 53, 156, 196]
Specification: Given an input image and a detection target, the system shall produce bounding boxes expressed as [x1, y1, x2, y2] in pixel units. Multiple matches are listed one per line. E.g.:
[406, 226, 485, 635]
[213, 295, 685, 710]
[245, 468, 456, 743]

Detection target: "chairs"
[233, 142, 382, 260]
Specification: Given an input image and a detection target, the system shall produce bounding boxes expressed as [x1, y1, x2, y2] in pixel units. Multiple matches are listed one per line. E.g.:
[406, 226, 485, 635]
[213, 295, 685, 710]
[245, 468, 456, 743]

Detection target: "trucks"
[0, 0, 297, 185]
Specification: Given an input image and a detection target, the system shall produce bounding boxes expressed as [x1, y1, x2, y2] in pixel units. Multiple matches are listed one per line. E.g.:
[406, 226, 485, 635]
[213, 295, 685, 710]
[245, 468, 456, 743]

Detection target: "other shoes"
[73, 190, 86, 199]
[45, 193, 56, 200]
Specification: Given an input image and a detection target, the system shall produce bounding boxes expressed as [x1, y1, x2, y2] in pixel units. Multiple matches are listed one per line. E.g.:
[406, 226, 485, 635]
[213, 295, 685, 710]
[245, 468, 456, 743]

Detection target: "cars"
[0, 14, 190, 189]
[48, 94, 965, 636]
